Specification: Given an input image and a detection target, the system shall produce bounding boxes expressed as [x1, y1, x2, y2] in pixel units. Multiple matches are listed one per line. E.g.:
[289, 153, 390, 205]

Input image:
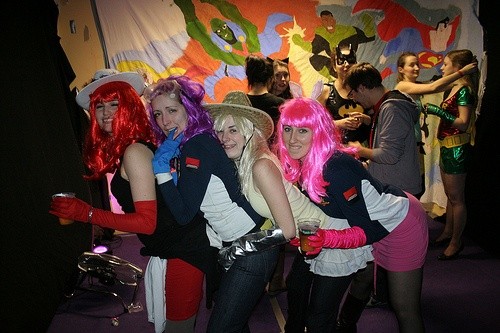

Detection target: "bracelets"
[457, 70, 464, 77]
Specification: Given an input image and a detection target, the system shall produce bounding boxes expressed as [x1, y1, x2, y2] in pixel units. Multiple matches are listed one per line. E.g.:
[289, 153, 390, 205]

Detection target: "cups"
[349, 113, 360, 120]
[52, 192, 76, 225]
[296, 217, 321, 252]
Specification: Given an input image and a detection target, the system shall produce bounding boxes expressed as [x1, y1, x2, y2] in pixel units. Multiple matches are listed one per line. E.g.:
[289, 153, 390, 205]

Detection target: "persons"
[142, 75, 279, 333]
[50, 70, 223, 333]
[201, 92, 376, 332]
[243, 53, 294, 145]
[391, 48, 478, 112]
[343, 62, 424, 199]
[424, 50, 481, 262]
[316, 44, 377, 164]
[274, 79, 430, 333]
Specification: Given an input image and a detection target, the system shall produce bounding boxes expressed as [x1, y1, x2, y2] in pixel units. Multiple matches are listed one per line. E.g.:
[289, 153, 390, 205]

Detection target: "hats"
[76, 69, 145, 110]
[202, 90, 274, 140]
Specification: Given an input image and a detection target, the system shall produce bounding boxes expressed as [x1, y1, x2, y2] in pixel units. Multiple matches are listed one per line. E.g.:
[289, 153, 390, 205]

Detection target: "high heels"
[432, 235, 452, 243]
[437, 242, 464, 260]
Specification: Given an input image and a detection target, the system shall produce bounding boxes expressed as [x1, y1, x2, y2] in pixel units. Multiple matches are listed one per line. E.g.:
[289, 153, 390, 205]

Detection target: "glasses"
[347, 88, 354, 99]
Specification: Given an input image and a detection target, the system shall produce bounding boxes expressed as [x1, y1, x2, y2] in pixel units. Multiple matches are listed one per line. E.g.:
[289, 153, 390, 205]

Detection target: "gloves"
[427, 104, 456, 124]
[151, 129, 184, 175]
[218, 225, 290, 271]
[49, 197, 157, 235]
[306, 227, 366, 255]
